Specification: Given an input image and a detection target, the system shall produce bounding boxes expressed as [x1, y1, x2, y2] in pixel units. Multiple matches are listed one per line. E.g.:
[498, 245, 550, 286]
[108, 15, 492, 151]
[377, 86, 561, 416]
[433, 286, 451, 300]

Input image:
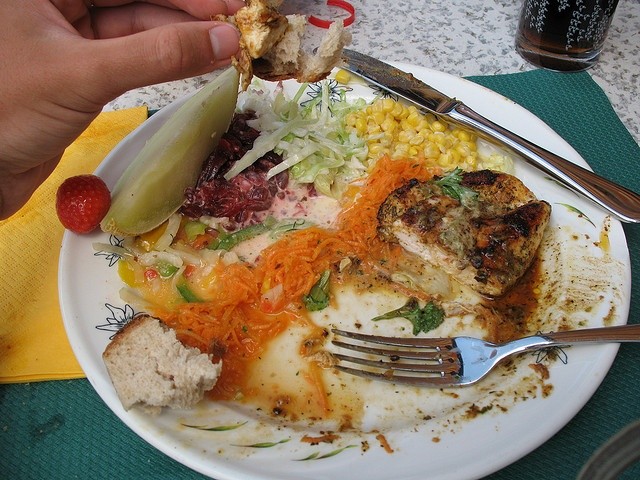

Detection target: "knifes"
[337, 47, 640, 225]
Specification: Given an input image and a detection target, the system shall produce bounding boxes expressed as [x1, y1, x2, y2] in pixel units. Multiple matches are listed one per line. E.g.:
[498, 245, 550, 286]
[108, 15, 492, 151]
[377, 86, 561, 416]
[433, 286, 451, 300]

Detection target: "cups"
[514, 1, 620, 74]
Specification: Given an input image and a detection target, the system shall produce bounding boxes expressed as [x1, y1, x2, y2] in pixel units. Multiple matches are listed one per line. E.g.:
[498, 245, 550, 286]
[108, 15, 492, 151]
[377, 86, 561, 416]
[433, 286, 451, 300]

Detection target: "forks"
[331, 323, 640, 389]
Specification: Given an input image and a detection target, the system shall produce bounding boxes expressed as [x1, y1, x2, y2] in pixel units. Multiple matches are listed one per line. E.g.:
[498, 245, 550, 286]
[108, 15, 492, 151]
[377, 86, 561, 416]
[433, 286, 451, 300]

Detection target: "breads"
[211, 0, 352, 93]
[102, 314, 227, 417]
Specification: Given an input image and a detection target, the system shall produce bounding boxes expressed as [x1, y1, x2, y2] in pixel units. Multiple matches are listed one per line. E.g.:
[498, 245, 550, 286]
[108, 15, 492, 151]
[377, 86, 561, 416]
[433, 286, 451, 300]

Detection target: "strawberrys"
[56, 173, 111, 234]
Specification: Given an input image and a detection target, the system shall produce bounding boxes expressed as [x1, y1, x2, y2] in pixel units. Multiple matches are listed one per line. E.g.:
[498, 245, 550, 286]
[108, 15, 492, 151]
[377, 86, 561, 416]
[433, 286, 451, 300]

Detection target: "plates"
[57, 60, 632, 480]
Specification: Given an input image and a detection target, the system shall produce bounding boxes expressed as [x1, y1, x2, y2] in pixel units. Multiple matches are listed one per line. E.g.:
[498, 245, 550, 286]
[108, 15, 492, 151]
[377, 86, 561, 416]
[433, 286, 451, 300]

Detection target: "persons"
[1, 1, 247, 220]
